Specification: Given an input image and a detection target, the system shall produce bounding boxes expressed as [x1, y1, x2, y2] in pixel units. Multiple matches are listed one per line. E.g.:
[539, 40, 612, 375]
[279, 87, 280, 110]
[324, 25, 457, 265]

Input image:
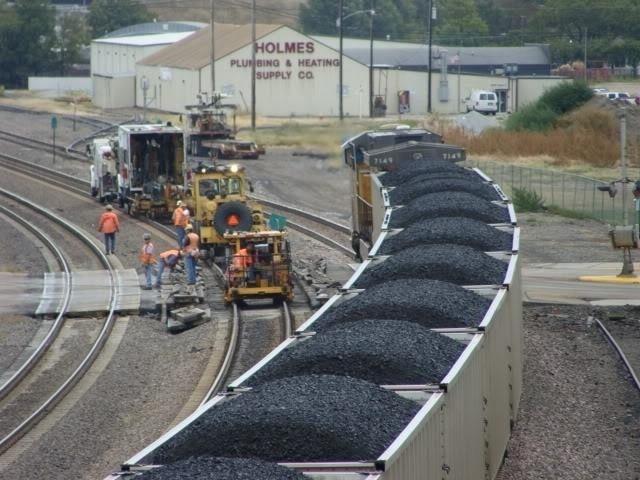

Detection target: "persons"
[170, 199, 199, 285]
[99, 204, 119, 256]
[224, 243, 254, 293]
[157, 250, 184, 287]
[139, 232, 155, 290]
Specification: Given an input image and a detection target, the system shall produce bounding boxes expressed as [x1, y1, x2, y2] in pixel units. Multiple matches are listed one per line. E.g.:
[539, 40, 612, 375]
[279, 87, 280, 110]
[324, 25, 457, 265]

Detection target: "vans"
[463, 88, 500, 117]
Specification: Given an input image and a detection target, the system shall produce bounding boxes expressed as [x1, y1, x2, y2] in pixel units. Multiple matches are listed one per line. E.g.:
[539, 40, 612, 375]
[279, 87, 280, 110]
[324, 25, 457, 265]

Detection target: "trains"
[98, 114, 535, 480]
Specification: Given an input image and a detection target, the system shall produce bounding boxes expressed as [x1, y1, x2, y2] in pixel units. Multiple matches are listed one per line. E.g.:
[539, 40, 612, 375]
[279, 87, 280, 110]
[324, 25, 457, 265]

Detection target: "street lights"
[139, 75, 152, 120]
[339, 8, 380, 121]
[502, 62, 520, 114]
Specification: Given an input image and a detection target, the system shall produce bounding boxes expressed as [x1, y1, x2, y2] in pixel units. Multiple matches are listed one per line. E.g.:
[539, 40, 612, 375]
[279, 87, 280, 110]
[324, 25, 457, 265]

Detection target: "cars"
[592, 87, 630, 99]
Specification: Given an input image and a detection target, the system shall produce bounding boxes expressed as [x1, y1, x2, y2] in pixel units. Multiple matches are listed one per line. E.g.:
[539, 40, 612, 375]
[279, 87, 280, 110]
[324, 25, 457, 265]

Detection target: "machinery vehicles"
[114, 122, 192, 222]
[81, 135, 121, 202]
[182, 90, 265, 161]
[179, 163, 272, 265]
[221, 222, 300, 310]
